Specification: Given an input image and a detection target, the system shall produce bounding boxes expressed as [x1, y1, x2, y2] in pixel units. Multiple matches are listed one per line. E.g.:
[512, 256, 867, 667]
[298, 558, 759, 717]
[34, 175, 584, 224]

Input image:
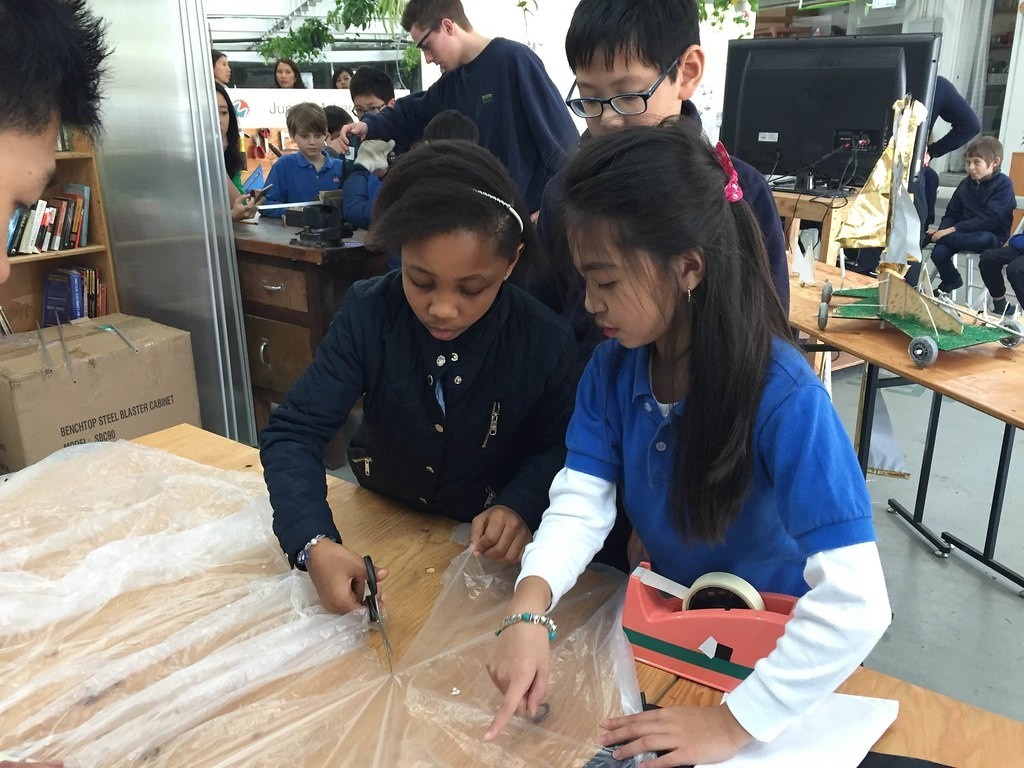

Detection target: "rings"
[247, 205, 251, 209]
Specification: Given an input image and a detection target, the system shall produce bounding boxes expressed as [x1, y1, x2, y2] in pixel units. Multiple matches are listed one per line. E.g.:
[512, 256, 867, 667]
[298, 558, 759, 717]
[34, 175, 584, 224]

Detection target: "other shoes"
[933, 276, 962, 297]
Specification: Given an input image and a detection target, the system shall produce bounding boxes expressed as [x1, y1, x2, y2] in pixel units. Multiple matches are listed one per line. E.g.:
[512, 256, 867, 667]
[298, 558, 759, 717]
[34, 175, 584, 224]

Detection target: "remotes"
[773, 178, 795, 184]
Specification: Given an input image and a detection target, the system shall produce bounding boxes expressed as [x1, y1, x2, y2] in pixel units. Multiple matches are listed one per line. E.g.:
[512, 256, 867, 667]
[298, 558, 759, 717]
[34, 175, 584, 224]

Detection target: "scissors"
[249, 183, 273, 204]
[351, 554, 394, 673]
[259, 128, 271, 154]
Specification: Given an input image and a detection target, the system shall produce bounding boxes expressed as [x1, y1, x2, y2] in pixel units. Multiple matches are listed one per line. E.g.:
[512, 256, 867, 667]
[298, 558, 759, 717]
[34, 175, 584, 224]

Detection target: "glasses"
[415, 20, 454, 50]
[565, 58, 680, 118]
[352, 102, 389, 118]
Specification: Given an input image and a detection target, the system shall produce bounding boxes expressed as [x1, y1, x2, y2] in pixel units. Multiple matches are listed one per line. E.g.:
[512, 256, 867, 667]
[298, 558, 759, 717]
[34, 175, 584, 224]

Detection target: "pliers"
[252, 134, 266, 159]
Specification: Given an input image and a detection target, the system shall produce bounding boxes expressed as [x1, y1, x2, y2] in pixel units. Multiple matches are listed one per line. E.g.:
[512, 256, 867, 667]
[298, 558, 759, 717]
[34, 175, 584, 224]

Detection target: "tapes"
[631, 566, 766, 611]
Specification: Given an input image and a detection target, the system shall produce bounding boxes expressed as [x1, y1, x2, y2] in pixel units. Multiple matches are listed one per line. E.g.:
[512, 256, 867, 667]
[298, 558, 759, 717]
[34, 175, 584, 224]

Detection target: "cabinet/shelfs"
[0, 125, 122, 333]
[232, 212, 389, 471]
[980, 7, 1018, 134]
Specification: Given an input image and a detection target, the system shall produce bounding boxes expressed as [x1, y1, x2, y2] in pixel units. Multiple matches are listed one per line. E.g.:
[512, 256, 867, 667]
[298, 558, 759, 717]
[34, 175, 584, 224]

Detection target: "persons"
[338, 0, 583, 223]
[843, 75, 980, 287]
[920, 134, 1017, 298]
[979, 213, 1024, 316]
[324, 105, 352, 161]
[339, 108, 480, 267]
[274, 58, 308, 90]
[350, 64, 396, 117]
[332, 65, 354, 90]
[484, 122, 894, 768]
[211, 48, 231, 88]
[528, 0, 790, 365]
[263, 141, 587, 621]
[214, 79, 267, 222]
[264, 103, 344, 217]
[0, 0, 115, 309]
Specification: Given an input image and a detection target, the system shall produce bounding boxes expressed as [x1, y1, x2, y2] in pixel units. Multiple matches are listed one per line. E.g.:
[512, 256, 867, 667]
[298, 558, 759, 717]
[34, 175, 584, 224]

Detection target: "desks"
[787, 254, 1024, 600]
[0, 422, 1024, 768]
[772, 186, 915, 376]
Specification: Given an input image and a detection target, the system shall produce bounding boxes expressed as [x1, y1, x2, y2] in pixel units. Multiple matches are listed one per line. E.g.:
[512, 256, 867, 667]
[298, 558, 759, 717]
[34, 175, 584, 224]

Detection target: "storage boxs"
[989, 73, 1008, 84]
[0, 310, 202, 473]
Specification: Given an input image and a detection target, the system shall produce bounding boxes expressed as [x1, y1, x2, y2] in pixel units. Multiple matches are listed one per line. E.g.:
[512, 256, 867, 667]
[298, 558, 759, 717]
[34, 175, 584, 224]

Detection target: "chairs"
[925, 196, 1024, 303]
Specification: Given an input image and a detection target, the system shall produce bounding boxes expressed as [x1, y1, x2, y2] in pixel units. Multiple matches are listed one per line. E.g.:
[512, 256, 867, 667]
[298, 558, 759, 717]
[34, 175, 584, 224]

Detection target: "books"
[0, 267, 107, 334]
[6, 180, 90, 254]
[55, 120, 74, 152]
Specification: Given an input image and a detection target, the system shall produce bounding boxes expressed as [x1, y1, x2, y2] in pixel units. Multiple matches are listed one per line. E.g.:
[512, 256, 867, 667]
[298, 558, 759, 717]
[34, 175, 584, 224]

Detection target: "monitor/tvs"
[720, 30, 943, 195]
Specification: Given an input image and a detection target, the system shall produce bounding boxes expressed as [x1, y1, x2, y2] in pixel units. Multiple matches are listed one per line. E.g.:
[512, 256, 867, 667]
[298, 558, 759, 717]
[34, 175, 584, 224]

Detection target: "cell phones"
[254, 184, 274, 204]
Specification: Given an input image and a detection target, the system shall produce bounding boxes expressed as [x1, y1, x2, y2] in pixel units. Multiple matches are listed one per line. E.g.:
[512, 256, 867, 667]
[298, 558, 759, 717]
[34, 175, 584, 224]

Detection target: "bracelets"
[296, 535, 338, 565]
[494, 613, 557, 641]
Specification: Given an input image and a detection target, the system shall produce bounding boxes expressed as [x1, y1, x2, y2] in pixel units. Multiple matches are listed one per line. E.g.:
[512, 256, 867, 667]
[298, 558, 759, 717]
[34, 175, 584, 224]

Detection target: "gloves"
[354, 139, 396, 172]
